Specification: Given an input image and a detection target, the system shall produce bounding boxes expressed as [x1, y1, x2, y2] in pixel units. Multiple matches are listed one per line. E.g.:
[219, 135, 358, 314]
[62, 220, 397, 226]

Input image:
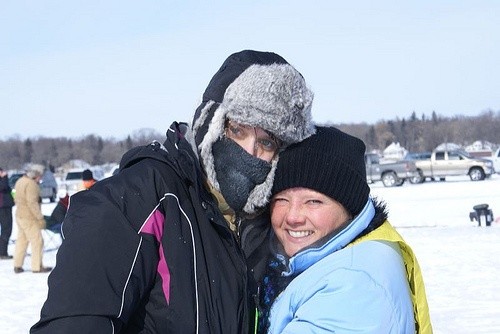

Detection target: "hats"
[183, 50, 317, 218]
[82, 169, 92, 180]
[269, 125, 370, 218]
[26, 163, 44, 178]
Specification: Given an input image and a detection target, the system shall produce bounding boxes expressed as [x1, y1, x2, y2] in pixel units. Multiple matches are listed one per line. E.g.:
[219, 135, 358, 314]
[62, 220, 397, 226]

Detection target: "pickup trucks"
[364, 153, 417, 188]
[407, 143, 495, 182]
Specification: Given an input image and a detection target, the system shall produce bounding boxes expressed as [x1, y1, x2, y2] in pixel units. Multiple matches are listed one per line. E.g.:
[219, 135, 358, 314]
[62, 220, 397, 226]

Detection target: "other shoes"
[0, 255, 12, 259]
[14, 267, 24, 273]
[33, 267, 52, 273]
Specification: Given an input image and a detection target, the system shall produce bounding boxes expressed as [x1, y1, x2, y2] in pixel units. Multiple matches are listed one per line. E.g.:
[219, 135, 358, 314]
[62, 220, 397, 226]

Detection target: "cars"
[7, 170, 58, 204]
[64, 170, 101, 195]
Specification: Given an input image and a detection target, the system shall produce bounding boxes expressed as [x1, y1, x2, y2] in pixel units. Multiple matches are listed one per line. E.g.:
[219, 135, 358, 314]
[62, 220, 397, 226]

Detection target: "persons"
[254, 125, 433, 334]
[13, 163, 52, 274]
[0, 167, 15, 259]
[28, 50, 317, 334]
[73, 168, 98, 193]
